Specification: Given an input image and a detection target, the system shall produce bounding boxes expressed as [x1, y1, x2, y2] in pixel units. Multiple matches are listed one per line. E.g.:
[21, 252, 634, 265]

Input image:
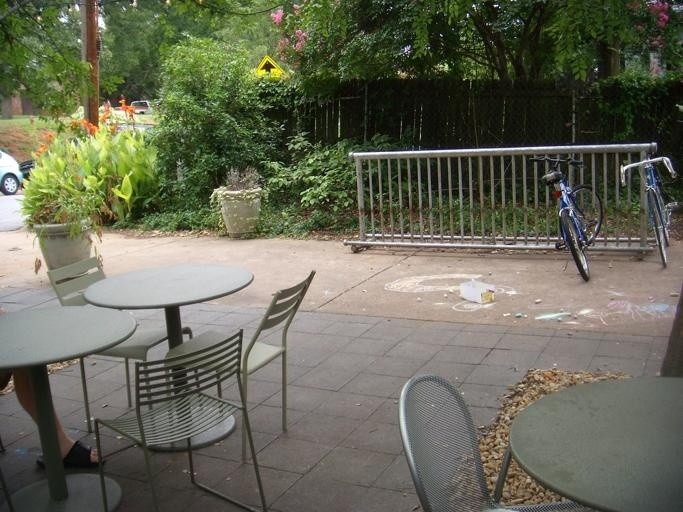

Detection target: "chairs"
[94, 329, 269, 512]
[48, 258, 203, 434]
[164, 270, 317, 458]
[399, 374, 591, 512]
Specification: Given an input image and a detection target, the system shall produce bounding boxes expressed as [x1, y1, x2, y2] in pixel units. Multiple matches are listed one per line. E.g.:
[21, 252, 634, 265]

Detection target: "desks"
[493, 377, 683, 512]
[84, 263, 254, 452]
[0, 306, 137, 512]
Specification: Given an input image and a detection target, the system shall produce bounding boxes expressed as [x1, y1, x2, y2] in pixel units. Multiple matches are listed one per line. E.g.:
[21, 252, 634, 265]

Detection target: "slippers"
[36, 440, 106, 467]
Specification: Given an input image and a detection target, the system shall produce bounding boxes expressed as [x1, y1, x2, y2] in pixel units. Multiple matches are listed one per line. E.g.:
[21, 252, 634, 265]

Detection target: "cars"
[130, 100, 154, 115]
[0, 151, 24, 196]
[18, 122, 157, 181]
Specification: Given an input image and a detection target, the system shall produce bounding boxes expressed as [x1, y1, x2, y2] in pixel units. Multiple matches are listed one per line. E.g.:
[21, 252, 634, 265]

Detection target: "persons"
[0, 363, 106, 468]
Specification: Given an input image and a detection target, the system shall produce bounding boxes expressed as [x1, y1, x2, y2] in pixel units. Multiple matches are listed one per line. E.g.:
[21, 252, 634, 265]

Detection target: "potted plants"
[31, 193, 94, 283]
[209, 185, 272, 238]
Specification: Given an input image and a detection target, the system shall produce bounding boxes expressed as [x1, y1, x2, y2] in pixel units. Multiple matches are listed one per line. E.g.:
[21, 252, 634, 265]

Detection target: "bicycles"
[528, 154, 603, 282]
[619, 156, 678, 268]
